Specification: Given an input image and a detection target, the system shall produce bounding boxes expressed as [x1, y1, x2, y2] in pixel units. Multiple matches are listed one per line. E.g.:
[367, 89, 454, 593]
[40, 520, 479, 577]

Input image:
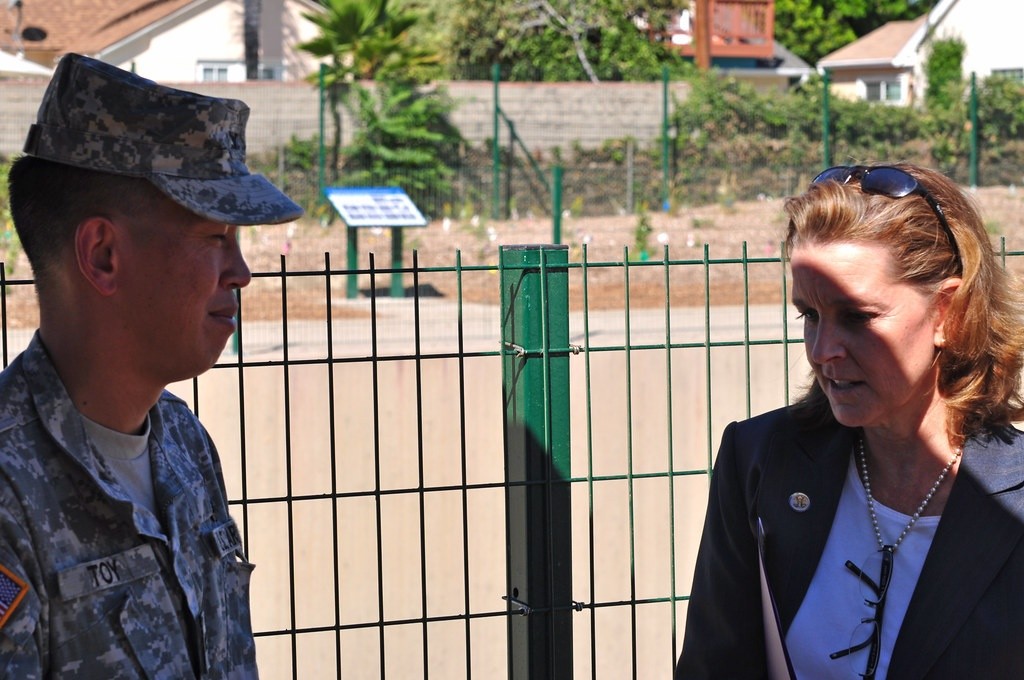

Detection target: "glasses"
[807, 166, 962, 275]
[829, 545, 893, 677]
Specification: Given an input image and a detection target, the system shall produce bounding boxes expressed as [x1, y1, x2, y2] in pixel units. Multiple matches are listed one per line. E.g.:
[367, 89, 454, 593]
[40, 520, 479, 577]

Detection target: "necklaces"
[857, 421, 961, 552]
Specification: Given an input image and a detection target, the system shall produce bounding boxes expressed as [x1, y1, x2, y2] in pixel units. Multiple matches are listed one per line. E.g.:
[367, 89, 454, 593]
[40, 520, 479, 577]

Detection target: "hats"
[22, 52, 302, 226]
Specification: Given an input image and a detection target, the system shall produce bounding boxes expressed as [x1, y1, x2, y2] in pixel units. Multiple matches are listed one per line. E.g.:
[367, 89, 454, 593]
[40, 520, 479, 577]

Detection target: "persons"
[672, 163, 1024, 680]
[0, 143, 262, 680]
